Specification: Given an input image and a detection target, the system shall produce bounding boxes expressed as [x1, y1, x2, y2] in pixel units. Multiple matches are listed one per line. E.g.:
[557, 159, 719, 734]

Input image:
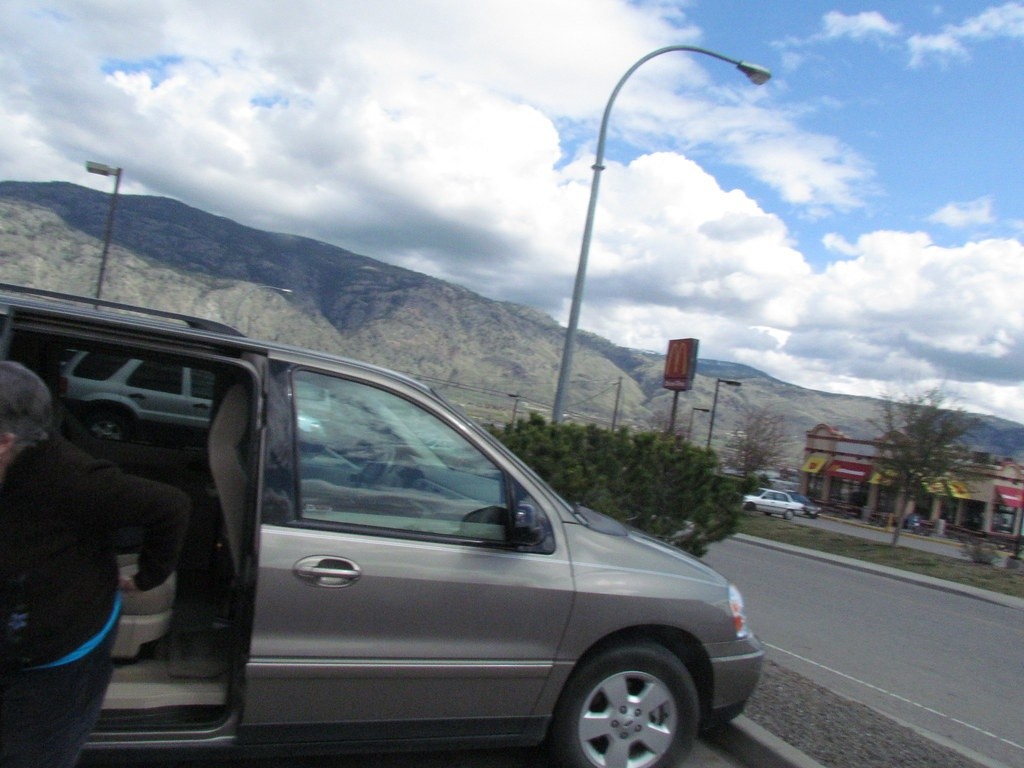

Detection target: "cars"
[778, 488, 822, 518]
[740, 486, 806, 521]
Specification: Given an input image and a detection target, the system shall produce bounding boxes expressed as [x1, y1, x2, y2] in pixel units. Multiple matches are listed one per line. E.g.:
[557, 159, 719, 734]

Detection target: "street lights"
[550, 44, 772, 436]
[507, 391, 520, 429]
[686, 404, 709, 442]
[84, 159, 122, 303]
[704, 378, 742, 449]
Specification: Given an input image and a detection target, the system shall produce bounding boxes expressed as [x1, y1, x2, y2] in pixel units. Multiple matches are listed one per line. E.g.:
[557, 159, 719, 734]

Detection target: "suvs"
[0, 284, 768, 768]
[53, 339, 329, 455]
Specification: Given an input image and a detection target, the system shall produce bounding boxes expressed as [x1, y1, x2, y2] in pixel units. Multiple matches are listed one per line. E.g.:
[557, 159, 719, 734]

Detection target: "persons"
[0, 361, 194, 768]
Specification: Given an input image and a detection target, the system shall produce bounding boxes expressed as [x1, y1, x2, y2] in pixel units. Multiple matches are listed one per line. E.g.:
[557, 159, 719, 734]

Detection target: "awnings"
[800, 456, 827, 473]
[824, 460, 874, 481]
[867, 468, 974, 500]
[995, 484, 1024, 508]
